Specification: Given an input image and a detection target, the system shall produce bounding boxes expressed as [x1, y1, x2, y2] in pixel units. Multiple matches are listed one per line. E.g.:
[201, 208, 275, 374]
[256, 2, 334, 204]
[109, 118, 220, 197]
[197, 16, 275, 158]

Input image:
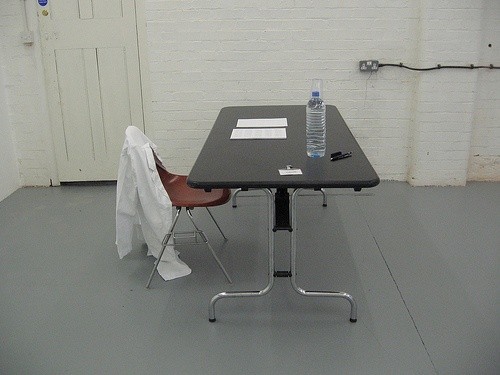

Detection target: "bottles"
[306, 91, 327, 157]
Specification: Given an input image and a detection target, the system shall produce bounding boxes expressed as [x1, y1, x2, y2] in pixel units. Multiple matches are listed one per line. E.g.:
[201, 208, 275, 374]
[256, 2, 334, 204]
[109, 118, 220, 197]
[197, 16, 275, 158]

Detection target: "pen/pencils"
[330, 152, 352, 161]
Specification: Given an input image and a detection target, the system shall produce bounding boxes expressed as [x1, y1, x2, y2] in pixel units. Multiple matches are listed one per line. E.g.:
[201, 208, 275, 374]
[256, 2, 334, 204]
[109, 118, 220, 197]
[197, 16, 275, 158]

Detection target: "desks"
[185, 104, 380, 322]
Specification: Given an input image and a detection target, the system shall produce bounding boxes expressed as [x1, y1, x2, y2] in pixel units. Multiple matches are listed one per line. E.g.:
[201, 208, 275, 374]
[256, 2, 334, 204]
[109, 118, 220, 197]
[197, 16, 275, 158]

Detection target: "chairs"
[124, 126, 233, 288]
